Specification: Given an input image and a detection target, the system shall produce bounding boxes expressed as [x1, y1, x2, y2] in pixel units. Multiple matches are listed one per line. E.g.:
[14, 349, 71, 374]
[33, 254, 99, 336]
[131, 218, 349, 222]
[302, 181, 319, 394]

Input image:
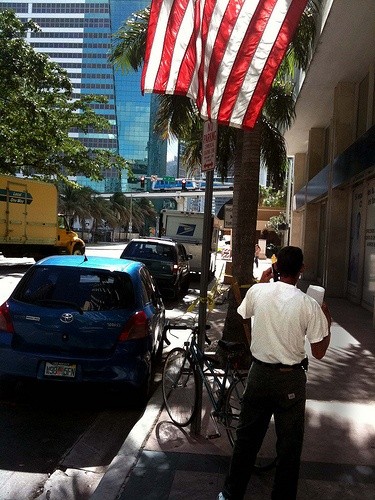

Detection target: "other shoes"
[216, 492, 226, 500]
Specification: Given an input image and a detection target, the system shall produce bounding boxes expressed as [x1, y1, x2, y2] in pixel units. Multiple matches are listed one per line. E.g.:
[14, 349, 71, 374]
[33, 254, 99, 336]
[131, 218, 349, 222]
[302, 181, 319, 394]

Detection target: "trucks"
[0, 174, 86, 263]
[158, 208, 224, 282]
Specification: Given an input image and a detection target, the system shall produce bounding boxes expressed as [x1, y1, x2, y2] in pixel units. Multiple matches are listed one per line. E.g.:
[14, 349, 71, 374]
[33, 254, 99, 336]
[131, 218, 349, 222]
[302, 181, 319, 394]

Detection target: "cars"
[0, 255, 168, 405]
[119, 236, 195, 301]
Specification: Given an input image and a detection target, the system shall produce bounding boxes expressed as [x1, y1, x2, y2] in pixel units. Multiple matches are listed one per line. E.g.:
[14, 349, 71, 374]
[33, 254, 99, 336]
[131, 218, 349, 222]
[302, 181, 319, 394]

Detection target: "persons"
[217, 247, 331, 500]
[256, 245, 260, 267]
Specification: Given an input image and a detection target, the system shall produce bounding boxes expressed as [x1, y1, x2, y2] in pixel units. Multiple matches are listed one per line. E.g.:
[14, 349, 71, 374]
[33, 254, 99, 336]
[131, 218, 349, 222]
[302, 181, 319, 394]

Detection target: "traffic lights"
[141, 176, 145, 188]
[181, 178, 187, 190]
[151, 175, 157, 182]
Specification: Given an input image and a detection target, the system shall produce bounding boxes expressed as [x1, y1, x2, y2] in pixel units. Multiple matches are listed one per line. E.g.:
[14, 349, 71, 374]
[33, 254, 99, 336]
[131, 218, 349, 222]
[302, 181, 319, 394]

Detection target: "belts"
[252, 357, 302, 371]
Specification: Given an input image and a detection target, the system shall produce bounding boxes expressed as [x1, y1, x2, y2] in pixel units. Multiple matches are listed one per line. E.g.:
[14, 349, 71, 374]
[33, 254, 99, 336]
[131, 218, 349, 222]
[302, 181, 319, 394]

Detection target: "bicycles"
[160, 323, 278, 473]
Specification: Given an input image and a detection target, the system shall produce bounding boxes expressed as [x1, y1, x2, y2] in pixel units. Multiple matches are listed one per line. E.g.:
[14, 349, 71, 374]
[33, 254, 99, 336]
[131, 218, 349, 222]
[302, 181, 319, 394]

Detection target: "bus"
[151, 176, 198, 191]
[200, 176, 235, 191]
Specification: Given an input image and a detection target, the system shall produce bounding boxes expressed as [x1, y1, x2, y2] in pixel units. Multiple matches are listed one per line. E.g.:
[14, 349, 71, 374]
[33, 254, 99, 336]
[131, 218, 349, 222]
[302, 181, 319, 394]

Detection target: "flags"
[141, 0, 309, 130]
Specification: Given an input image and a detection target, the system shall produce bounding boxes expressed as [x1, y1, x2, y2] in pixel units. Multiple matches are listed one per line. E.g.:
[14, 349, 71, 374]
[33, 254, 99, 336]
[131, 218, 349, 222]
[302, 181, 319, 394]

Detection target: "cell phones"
[272, 263, 278, 283]
